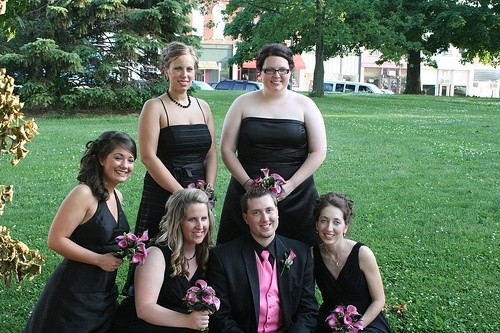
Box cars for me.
[215,80,260,91]
[191,80,214,91]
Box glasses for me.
[261,68,289,75]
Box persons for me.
[310,191,390,333]
[216,42,328,245]
[119,40,216,296]
[23,131,136,333]
[206,185,318,333]
[93,189,213,333]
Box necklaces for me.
[325,239,347,267]
[187,252,196,260]
[167,89,191,108]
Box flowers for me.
[250,168,287,199]
[279,248,297,277]
[187,179,218,209]
[181,279,221,332]
[324,304,364,333]
[115,229,149,266]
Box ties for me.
[261,250,272,277]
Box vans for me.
[322,81,395,95]
